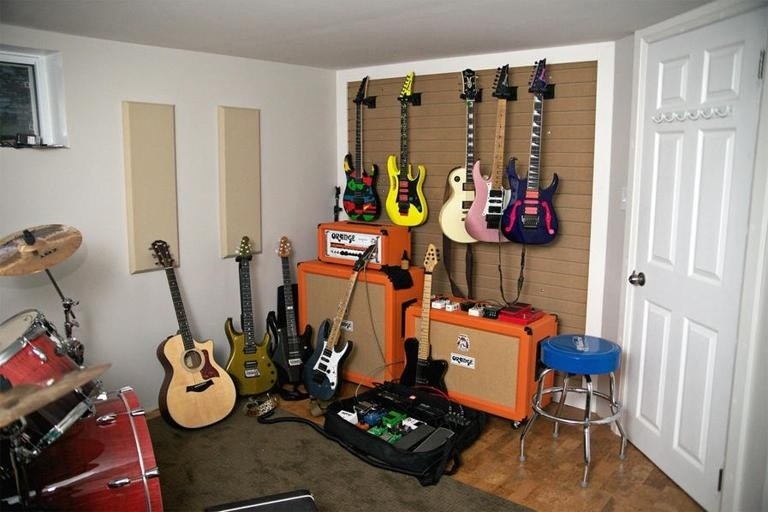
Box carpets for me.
[146,395,529,510]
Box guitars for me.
[343,73,382,222]
[265,235,316,385]
[225,236,279,399]
[149,238,238,430]
[302,246,376,402]
[438,68,489,244]
[384,71,430,229]
[398,243,448,394]
[500,58,559,245]
[464,63,512,244]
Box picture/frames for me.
[0,61,40,139]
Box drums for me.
[0,386,163,511]
[0,308,99,477]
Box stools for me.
[517,333,625,468]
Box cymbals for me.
[0,224,82,276]
[1,363,112,427]
